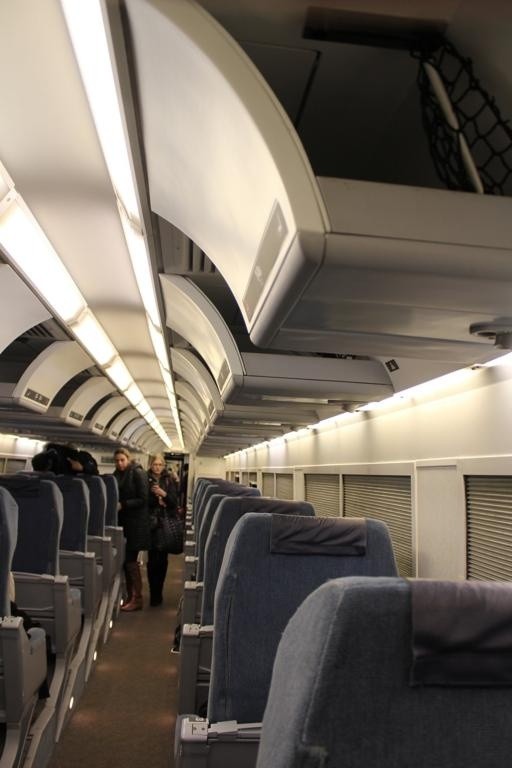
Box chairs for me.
[171,475,510,766]
[0,470,127,768]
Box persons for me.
[167,467,180,495]
[180,462,190,504]
[140,453,184,608]
[45,444,101,475]
[107,448,148,611]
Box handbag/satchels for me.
[160,505,184,555]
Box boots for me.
[121,560,144,611]
